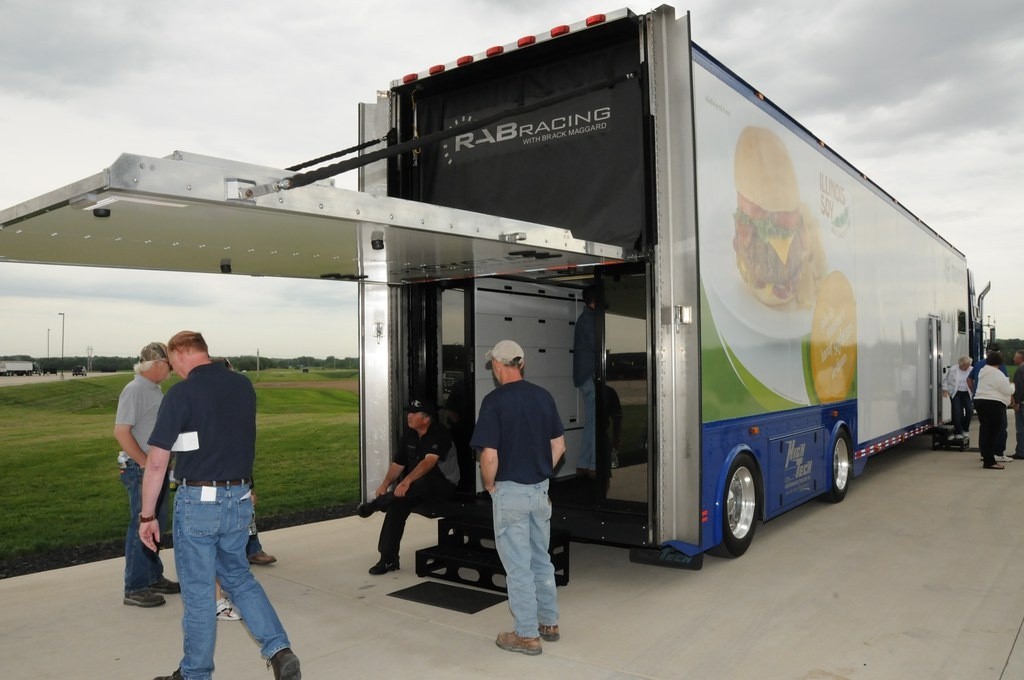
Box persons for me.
[572,287,595,471]
[1006,349,1024,459]
[139,331,302,680]
[115,342,181,607]
[966,343,1013,462]
[357,397,461,576]
[470,340,566,655]
[972,352,1015,470]
[941,356,973,439]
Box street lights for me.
[58,312,65,379]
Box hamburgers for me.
[733,127,803,304]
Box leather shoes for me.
[148,575,181,594]
[124,590,166,608]
[247,550,277,564]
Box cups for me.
[169,468,177,489]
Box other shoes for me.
[1008,454,1024,460]
[984,464,1004,469]
[981,456,983,460]
[995,455,1013,462]
[575,467,596,477]
[956,435,964,439]
[962,431,968,437]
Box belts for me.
[179,477,250,486]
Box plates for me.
[699,192,814,338]
[700,271,809,406]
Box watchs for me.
[139,512,156,523]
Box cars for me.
[72,366,88,378]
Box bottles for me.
[248,507,256,534]
[118,451,128,467]
[611,448,618,468]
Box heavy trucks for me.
[2,3,996,601]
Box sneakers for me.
[368,559,400,574]
[537,624,560,641]
[357,500,379,518]
[496,630,543,654]
[264,647,301,680]
[215,597,242,621]
[153,667,184,680]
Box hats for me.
[485,340,524,364]
[140,342,173,371]
[401,399,434,414]
